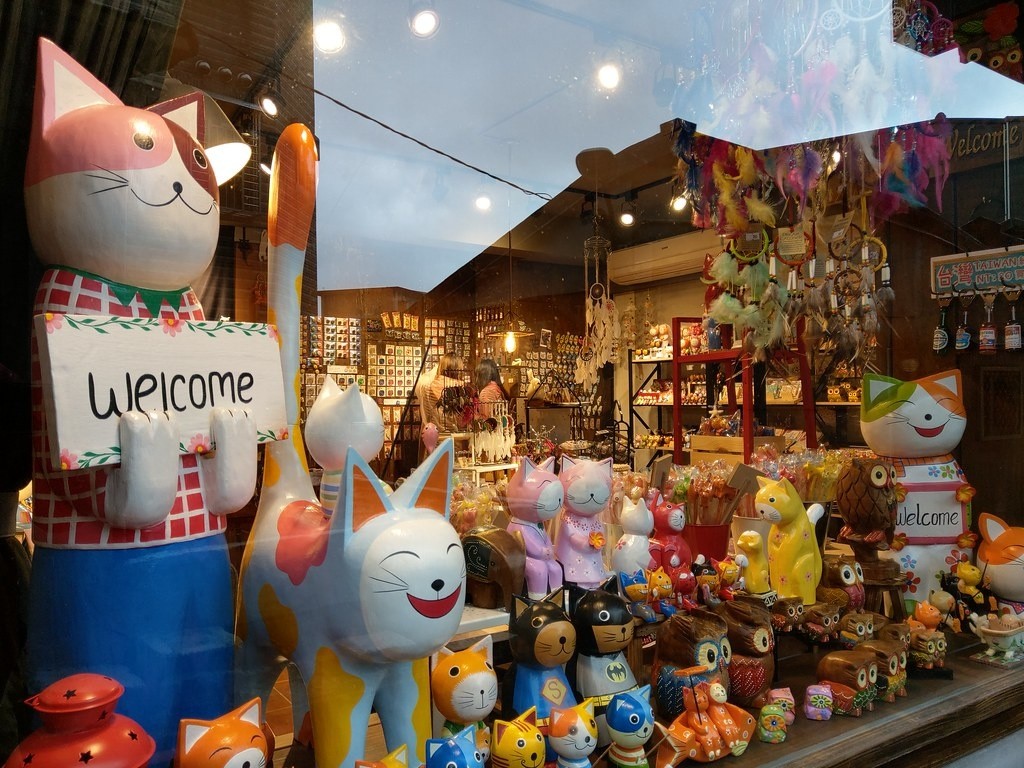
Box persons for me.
[475,357,511,433]
[414,352,466,464]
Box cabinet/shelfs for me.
[628,318,869,472]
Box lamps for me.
[671,179,686,210]
[620,191,638,227]
[407,0,442,40]
[651,54,677,106]
[485,141,536,353]
[580,192,596,224]
[258,49,287,121]
[595,29,625,89]
